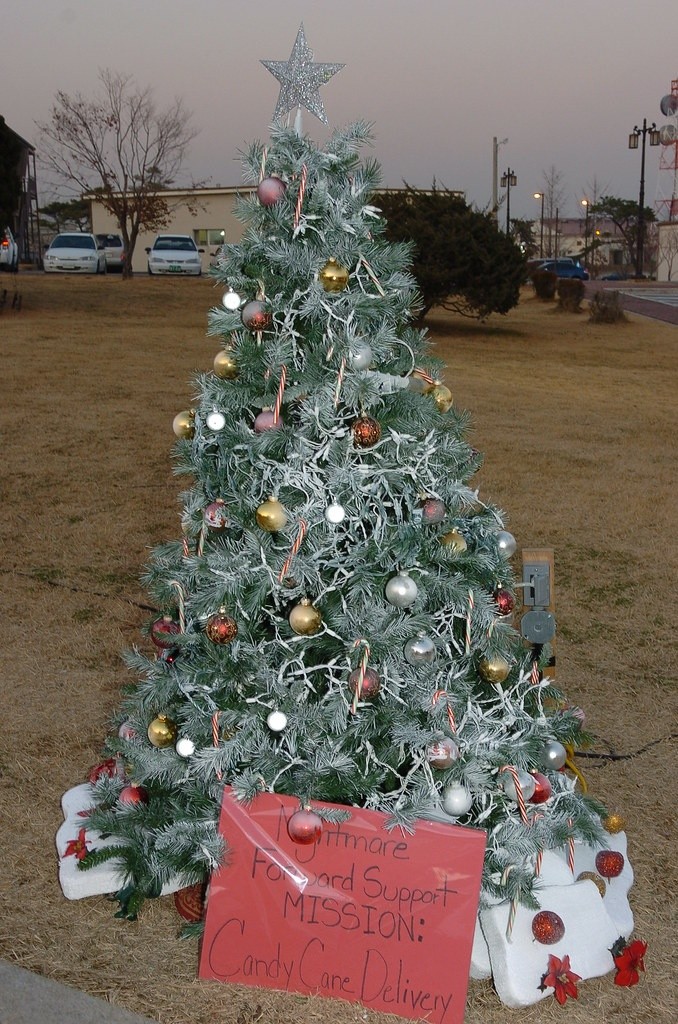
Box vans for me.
[526,258,575,272]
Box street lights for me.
[500,167,517,240]
[533,193,544,259]
[581,200,588,268]
[628,119,661,280]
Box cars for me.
[600,272,628,282]
[209,243,241,267]
[43,232,107,275]
[0,225,19,274]
[91,233,125,272]
[537,262,590,281]
[145,234,205,277]
